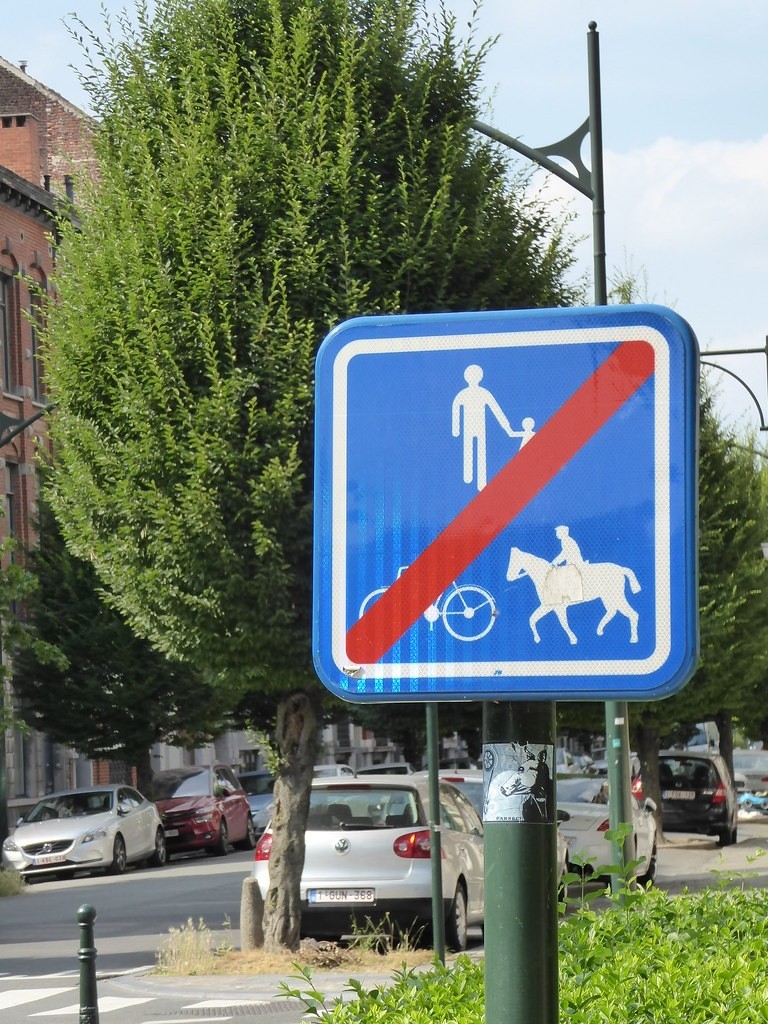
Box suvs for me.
[141,763,256,861]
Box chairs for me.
[322,804,353,830]
[658,764,673,786]
[692,766,711,788]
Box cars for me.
[2,784,166,883]
[556,747,594,774]
[686,724,715,752]
[355,762,414,776]
[632,751,745,845]
[252,774,483,952]
[629,751,641,777]
[733,750,768,814]
[421,757,475,770]
[238,770,275,839]
[313,764,354,777]
[556,773,658,889]
[381,769,570,903]
[589,759,608,773]
